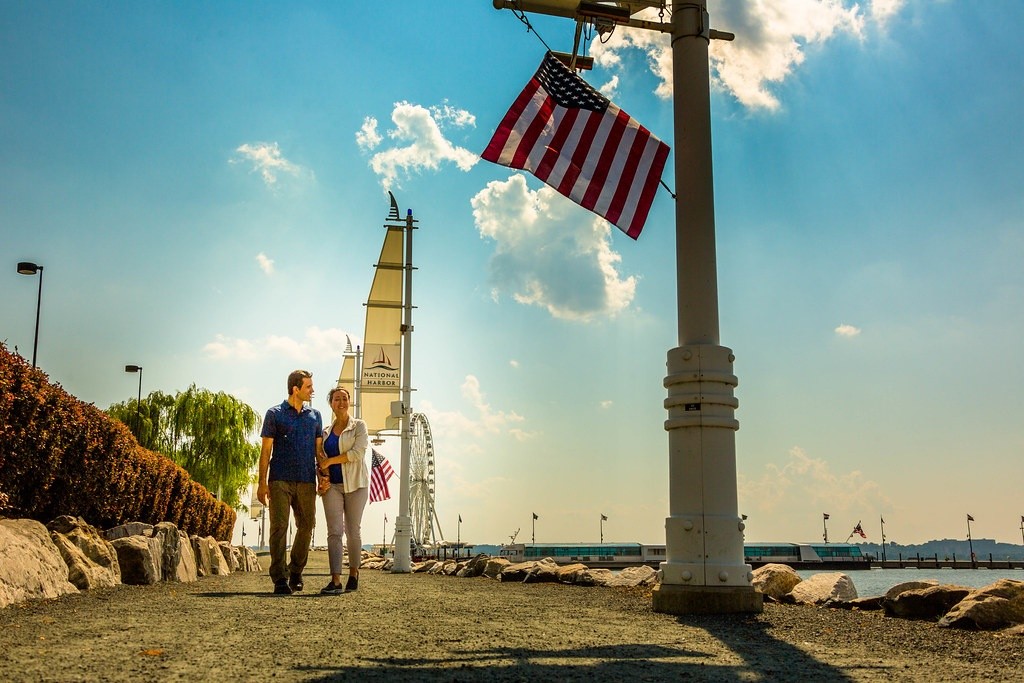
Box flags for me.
[881,518,884,523]
[855,525,866,538]
[459,516,462,522]
[601,515,607,521]
[385,517,388,522]
[534,514,538,520]
[370,450,394,504]
[742,515,747,520]
[824,514,829,519]
[481,51,670,240]
[968,515,973,521]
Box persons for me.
[257,371,329,594]
[316,387,369,594]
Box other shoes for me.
[289,569,304,591]
[345,569,359,592]
[274,582,293,594]
[321,581,342,594]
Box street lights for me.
[17,260,43,366]
[124,365,142,435]
